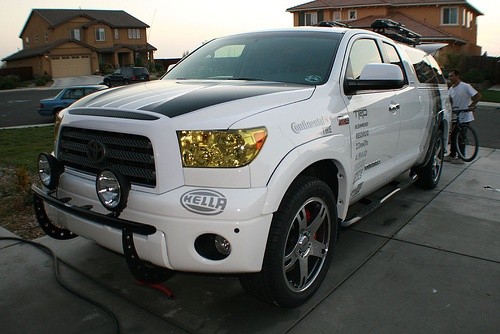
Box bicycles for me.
[451,107,479,163]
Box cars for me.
[37,84,109,122]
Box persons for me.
[442,69,481,164]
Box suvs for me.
[102,66,150,89]
[28,18,453,310]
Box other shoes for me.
[457,158,463,164]
[443,155,452,162]
